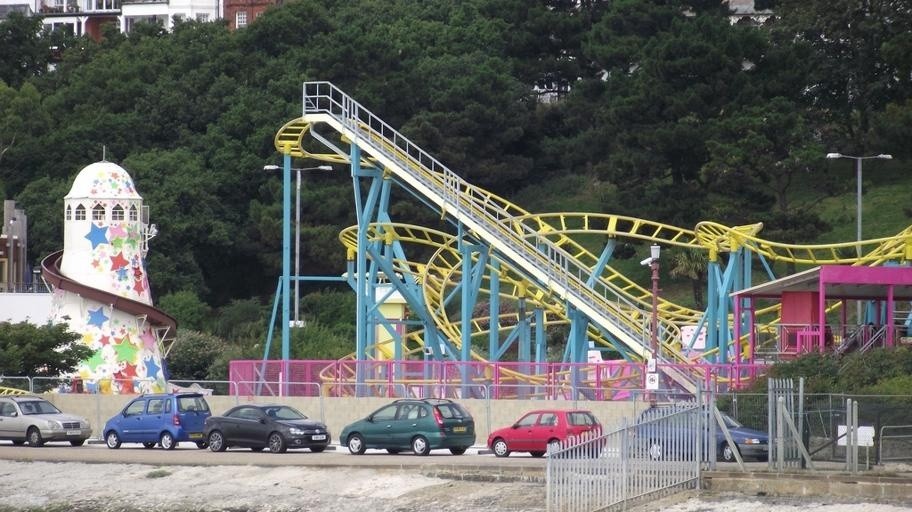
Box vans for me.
[101,390,219,449]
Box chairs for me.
[240,409,279,420]
[546,416,574,425]
[397,406,426,418]
[152,401,195,411]
[3,404,42,414]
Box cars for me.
[0,393,95,447]
[201,401,334,456]
[485,407,607,461]
[630,401,777,465]
[337,394,480,456]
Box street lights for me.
[259,162,335,329]
[825,151,896,326]
[647,241,665,408]
